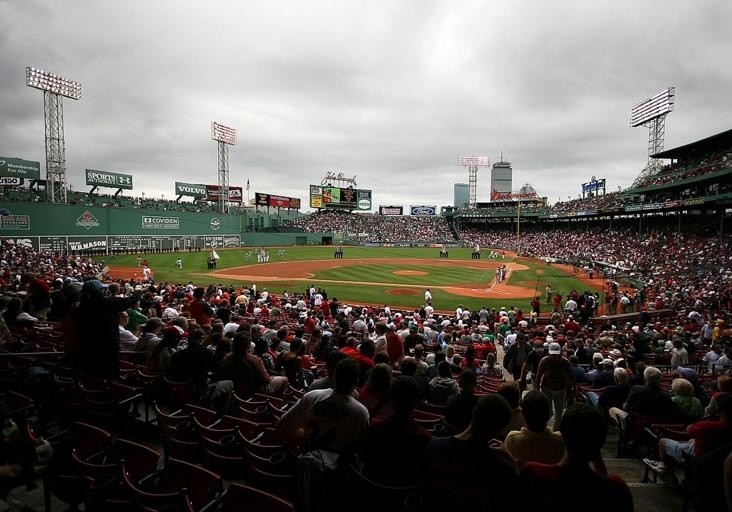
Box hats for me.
[548,342,561,355]
[610,349,621,356]
[599,358,614,366]
[83,279,110,292]
[677,365,696,380]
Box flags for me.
[246,180,250,189]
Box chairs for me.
[0,316,731,511]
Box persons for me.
[459,148,732,239]
[1,240,732,512]
[283,209,457,245]
